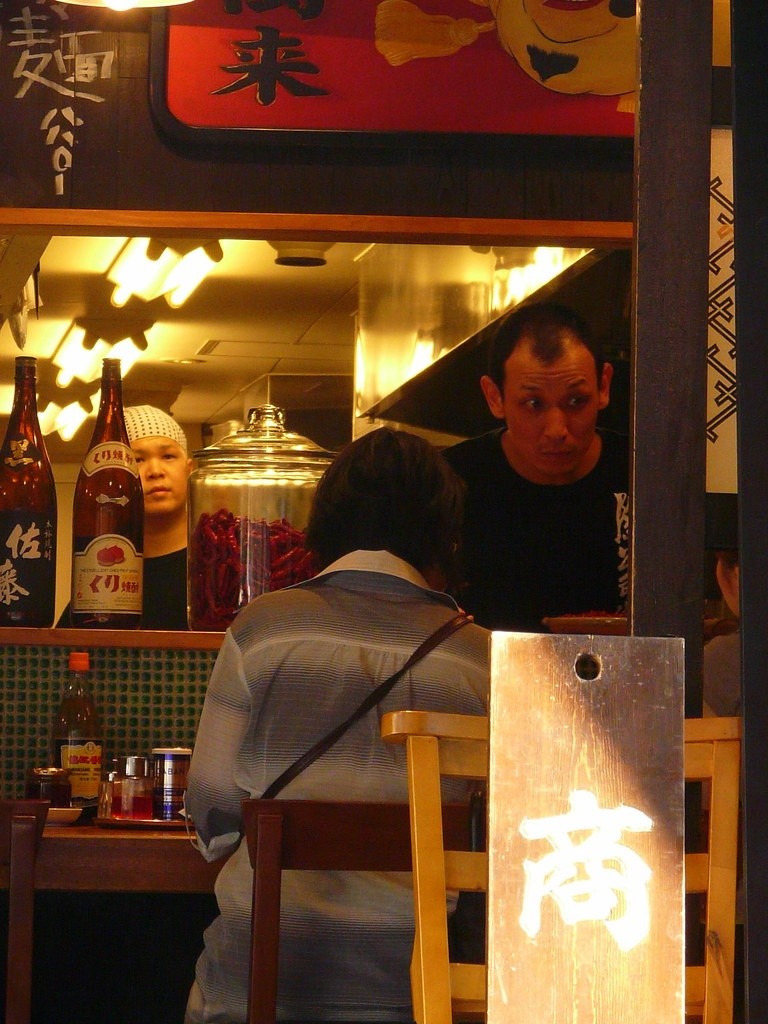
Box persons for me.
[431,298,636,643]
[114,400,201,626]
[698,527,746,730]
[185,422,505,1024]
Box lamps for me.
[29,236,225,441]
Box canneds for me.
[150,748,193,819]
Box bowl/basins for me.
[44,808,83,827]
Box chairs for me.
[239,793,472,1024]
[379,711,750,1024]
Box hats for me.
[124,403,188,452]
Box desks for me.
[31,825,245,895]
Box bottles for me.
[51,650,102,826]
[186,402,340,633]
[152,747,191,820]
[72,358,144,631]
[111,756,156,822]
[25,766,72,809]
[97,772,118,818]
[2,355,58,627]
